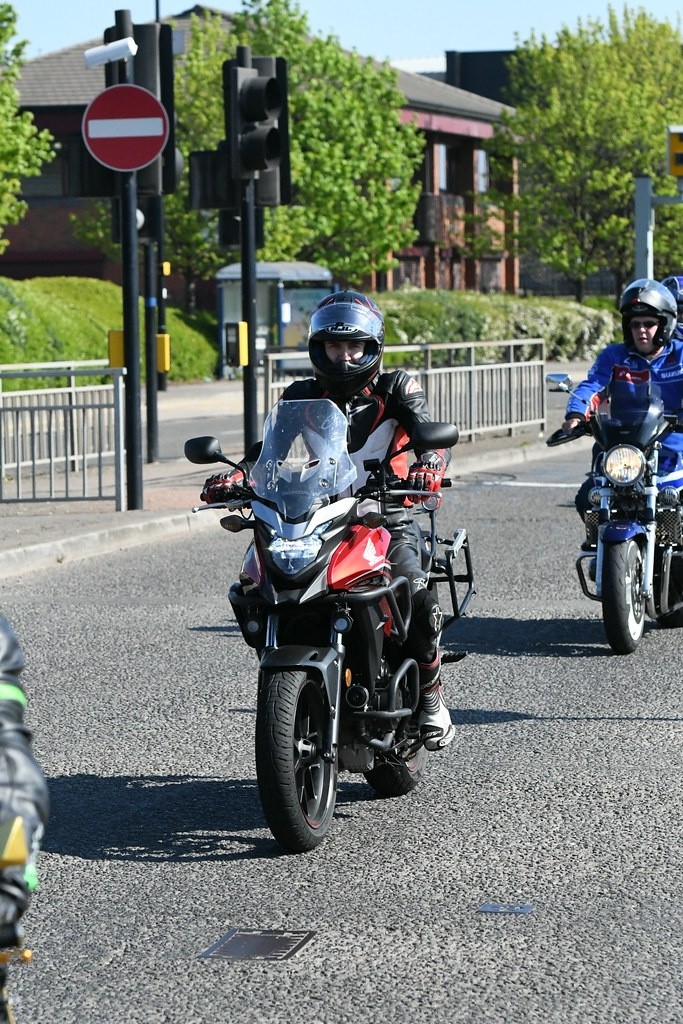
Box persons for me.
[563,274,683,555]
[202,289,458,752]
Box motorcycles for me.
[183,394,477,853]
[544,373,683,654]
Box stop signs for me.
[82,83,170,172]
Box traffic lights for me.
[230,66,285,181]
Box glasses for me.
[627,320,660,329]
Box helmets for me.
[621,279,677,350]
[307,290,384,398]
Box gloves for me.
[407,447,451,503]
[201,463,253,509]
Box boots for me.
[417,648,454,750]
